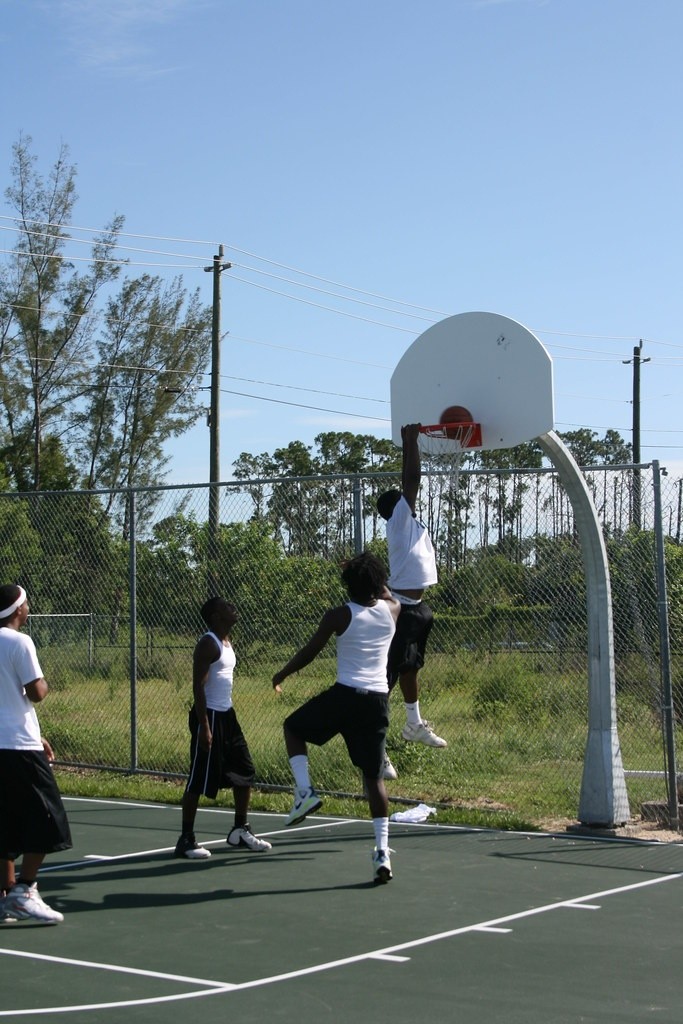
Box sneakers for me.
[227,823,272,851]
[402,719,446,748]
[175,832,211,858]
[284,786,322,825]
[371,845,396,882]
[2,882,64,922]
[383,752,398,781]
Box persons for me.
[174,597,272,858]
[377,423,448,779]
[0,583,72,923]
[272,551,401,883]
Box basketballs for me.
[439,406,474,440]
